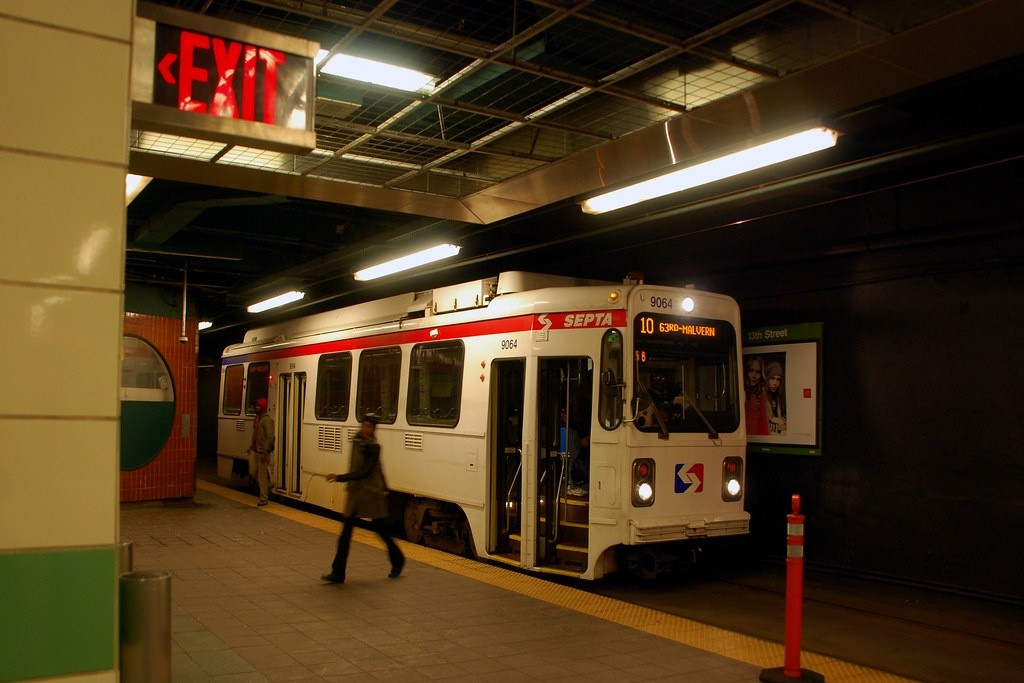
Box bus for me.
[217,271,754,581]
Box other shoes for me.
[257,500,267,506]
[388,560,406,578]
[320,573,345,584]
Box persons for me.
[648,368,681,413]
[568,368,593,496]
[744,356,787,435]
[320,417,405,584]
[505,364,561,444]
[246,398,275,505]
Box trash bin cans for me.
[119,538,135,575]
[119,569,173,683]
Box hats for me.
[763,361,782,380]
[256,398,268,414]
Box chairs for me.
[333,395,349,408]
